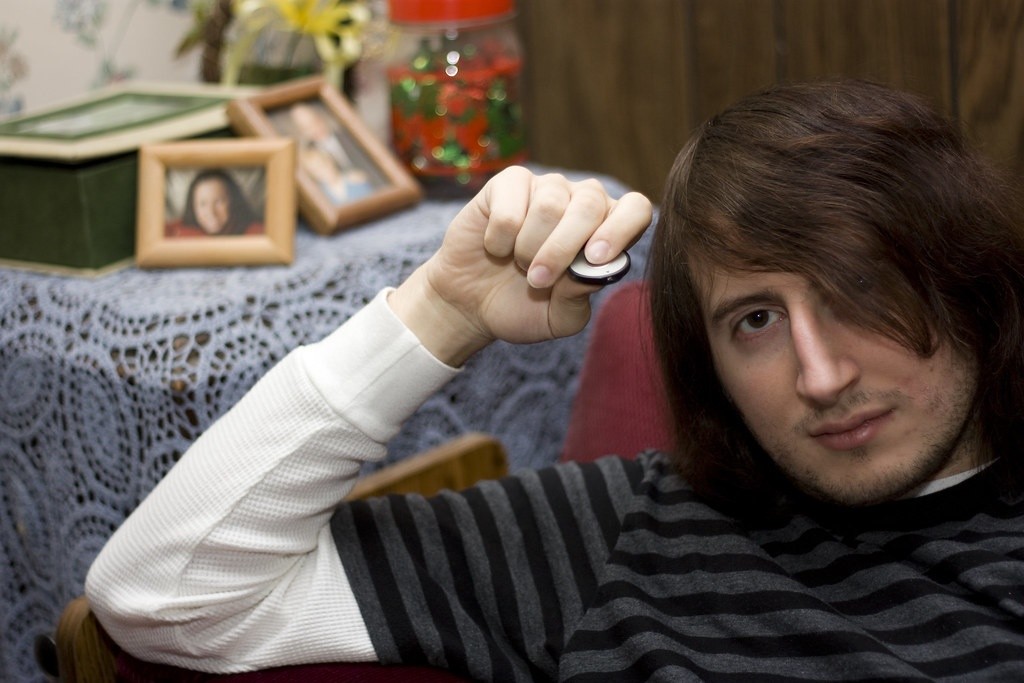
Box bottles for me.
[386,0,526,195]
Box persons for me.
[294,103,380,205]
[82,76,1024,683]
[167,171,263,237]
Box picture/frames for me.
[229,75,421,235]
[137,137,298,268]
[0,78,258,159]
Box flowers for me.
[177,1,370,68]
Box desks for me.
[1,156,659,683]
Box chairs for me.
[36,431,512,683]
